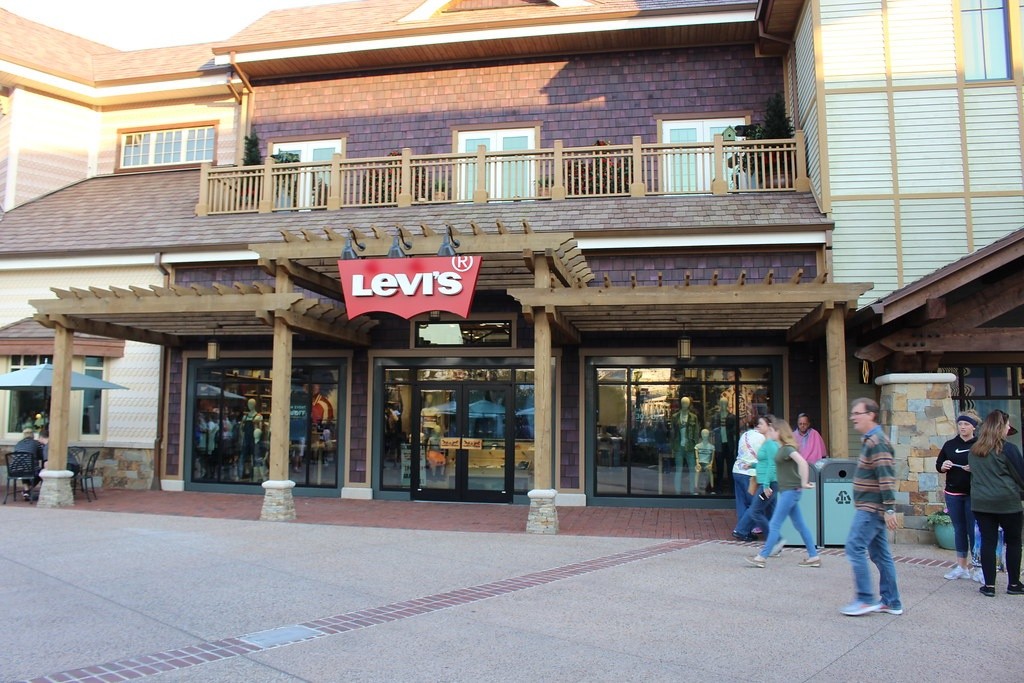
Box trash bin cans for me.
[777,461,820,548]
[815,457,858,548]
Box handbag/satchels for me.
[747,477,759,495]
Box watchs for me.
[886,509,894,514]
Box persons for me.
[239,398,263,477]
[38,430,80,478]
[968,410,1024,597]
[840,398,903,615]
[732,414,786,556]
[383,403,403,468]
[743,419,821,567]
[12,428,43,500]
[670,397,700,495]
[790,413,827,465]
[710,397,738,494]
[427,424,446,481]
[693,429,716,495]
[196,411,241,480]
[936,409,987,584]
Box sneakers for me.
[973,568,985,585]
[875,603,903,614]
[840,600,880,615]
[945,565,970,580]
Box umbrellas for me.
[196,383,246,399]
[0,357,130,431]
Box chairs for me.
[3,446,100,504]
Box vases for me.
[935,523,956,550]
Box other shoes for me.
[732,530,758,542]
[773,538,785,556]
[752,528,762,533]
[979,586,995,596]
[22,491,30,500]
[1007,581,1024,594]
[745,557,764,568]
[799,558,820,566]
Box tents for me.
[423,399,535,439]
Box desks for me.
[659,450,676,495]
[288,443,336,485]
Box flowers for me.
[925,507,953,527]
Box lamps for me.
[428,309,440,321]
[438,225,461,256]
[677,322,690,361]
[341,229,366,260]
[206,329,219,362]
[388,227,413,257]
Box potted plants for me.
[738,93,796,187]
[240,123,262,211]
[271,148,299,211]
[429,180,448,201]
[532,177,554,198]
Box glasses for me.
[849,411,868,416]
